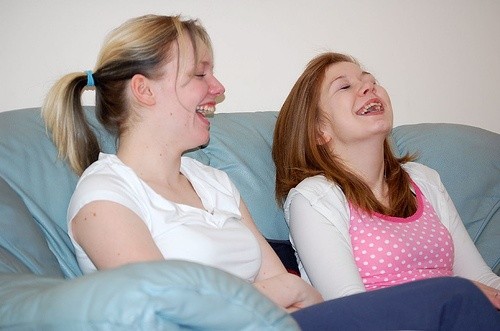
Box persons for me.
[41,14,500,331]
[271,51,500,315]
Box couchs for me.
[0,106,500,331]
